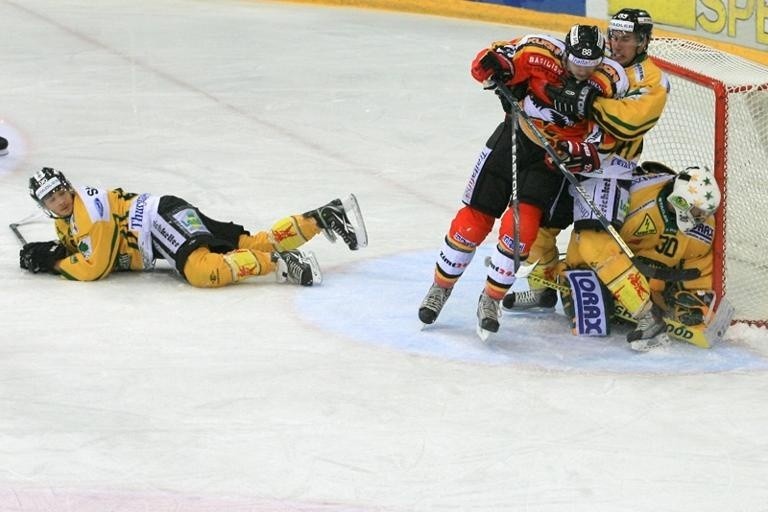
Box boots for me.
[271,249,312,286]
[303,199,358,250]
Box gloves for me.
[471,48,515,89]
[545,74,602,120]
[544,140,600,172]
[20,241,65,273]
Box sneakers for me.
[627,310,665,342]
[419,282,453,324]
[477,289,502,332]
[503,288,557,309]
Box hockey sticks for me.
[497,76,700,281]
[484,257,734,347]
[10,220,37,248]
[511,95,540,279]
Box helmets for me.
[607,8,653,47]
[565,24,605,72]
[29,167,75,219]
[666,165,721,233]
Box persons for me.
[503,7,670,312]
[553,162,723,352]
[419,25,629,340]
[0,137,9,156]
[18,166,368,290]
